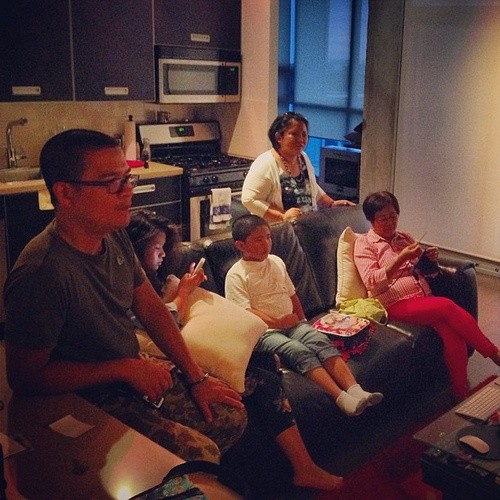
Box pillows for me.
[335,226,367,306]
[133,274,272,392]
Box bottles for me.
[125,115,136,160]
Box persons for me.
[354,191,500,396]
[225,214,383,416]
[2,128,247,464]
[242,111,358,220]
[125,208,344,490]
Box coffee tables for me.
[411,376,500,500]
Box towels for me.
[208,187,232,230]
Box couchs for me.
[164,203,480,490]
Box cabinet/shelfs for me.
[0,0,242,101]
[1,192,56,276]
[132,175,182,238]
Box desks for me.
[0,343,247,500]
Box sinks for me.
[0,168,43,183]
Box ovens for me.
[179,190,251,248]
[156,57,242,104]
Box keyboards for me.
[453,386,500,424]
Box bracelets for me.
[329,201,333,208]
[192,370,208,385]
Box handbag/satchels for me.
[338,298,389,326]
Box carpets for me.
[310,374,498,500]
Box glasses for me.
[146,366,184,408]
[64,174,139,193]
[275,112,303,132]
[373,214,399,226]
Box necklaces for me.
[281,156,303,182]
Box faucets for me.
[5,116,29,169]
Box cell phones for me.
[192,258,205,275]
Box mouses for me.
[458,435,489,454]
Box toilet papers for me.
[122,120,136,160]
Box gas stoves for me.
[136,121,255,194]
